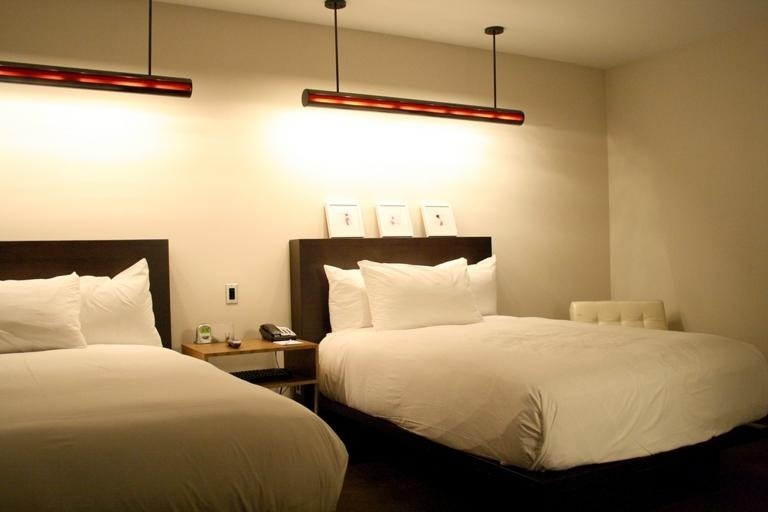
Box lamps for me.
[301,0,525,127]
[0,0,194,99]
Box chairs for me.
[568,300,668,331]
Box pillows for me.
[322,263,373,335]
[0,270,87,354]
[78,257,163,347]
[467,254,498,318]
[356,257,482,336]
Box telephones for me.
[259,323,297,343]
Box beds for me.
[0,238,351,512]
[289,235,768,512]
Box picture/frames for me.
[375,204,413,238]
[324,203,365,237]
[420,205,458,236]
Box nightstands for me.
[181,338,322,416]
